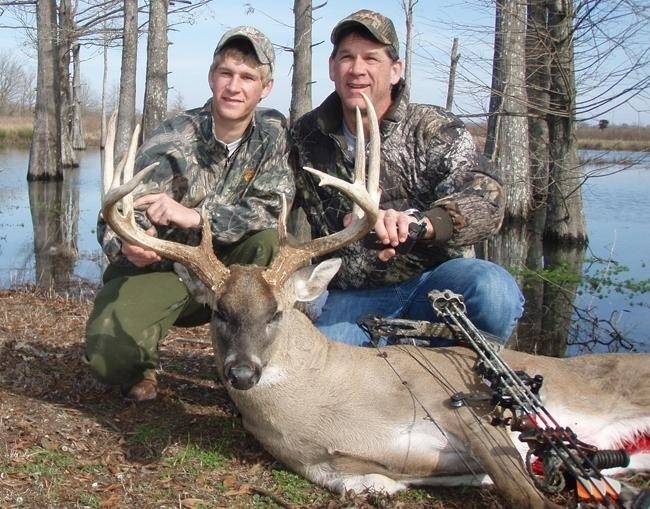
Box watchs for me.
[404,208,427,238]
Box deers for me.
[100,90,650,502]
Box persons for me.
[289,9,525,349]
[81,26,297,402]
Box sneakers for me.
[120,369,159,401]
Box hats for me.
[331,9,399,53]
[214,26,275,65]
